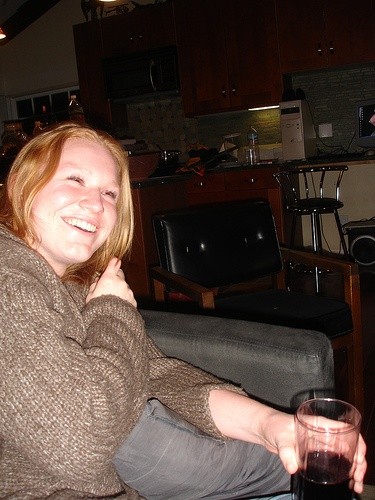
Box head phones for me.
[281,88,305,102]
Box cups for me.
[290,398,362,500]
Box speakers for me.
[346,228,375,292]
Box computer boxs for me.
[279,100,312,162]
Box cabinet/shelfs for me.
[72,0,375,309]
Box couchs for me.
[139,310,335,420]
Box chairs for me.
[150,197,365,420]
[274,166,350,261]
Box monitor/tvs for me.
[355,98,375,156]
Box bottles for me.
[246,125,260,166]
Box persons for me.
[0,122,367,500]
